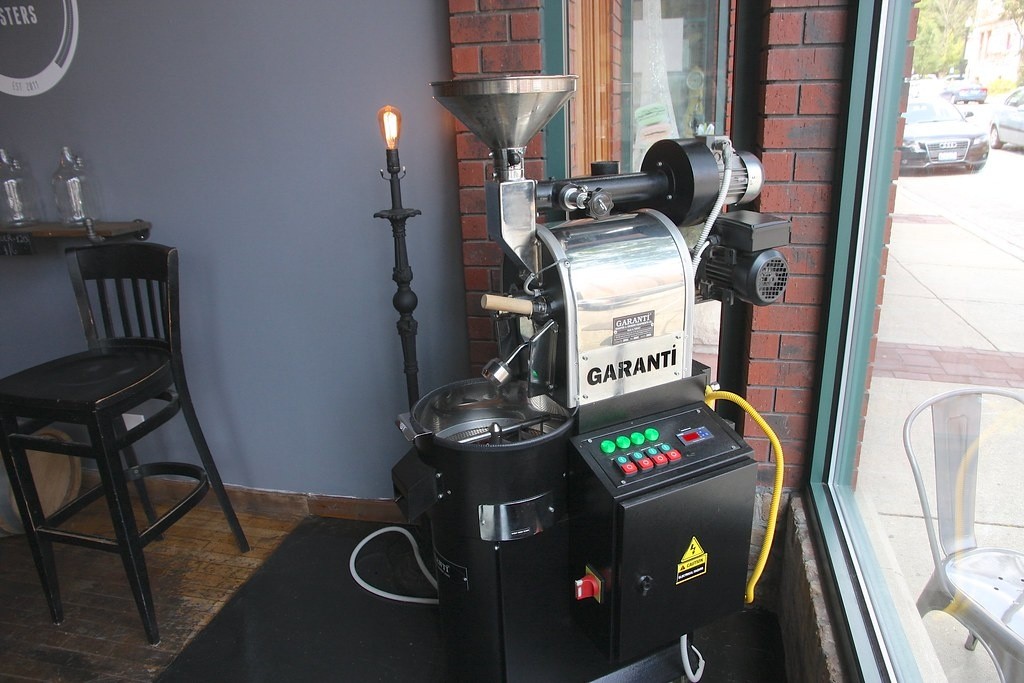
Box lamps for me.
[374,107,437,596]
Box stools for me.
[0,240,250,647]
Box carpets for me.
[156,514,788,683]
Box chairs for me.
[902,385,1024,683]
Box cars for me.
[900,75,989,171]
[989,85,1024,149]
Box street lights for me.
[959,16,973,80]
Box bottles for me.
[0,148,42,229]
[49,145,99,228]
